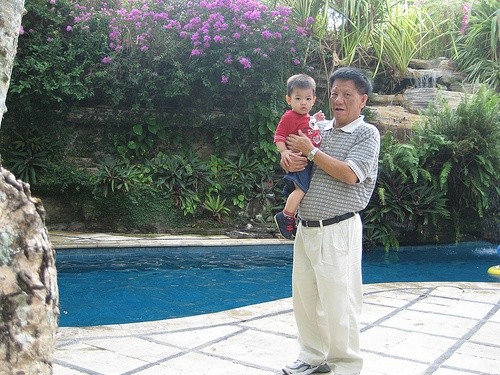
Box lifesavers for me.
[487,264,500,275]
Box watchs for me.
[306,147,321,162]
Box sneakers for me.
[282,359,332,375]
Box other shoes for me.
[292,216,298,238]
[274,212,294,240]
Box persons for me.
[273,73,328,242]
[282,67,381,374]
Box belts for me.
[295,212,358,227]
